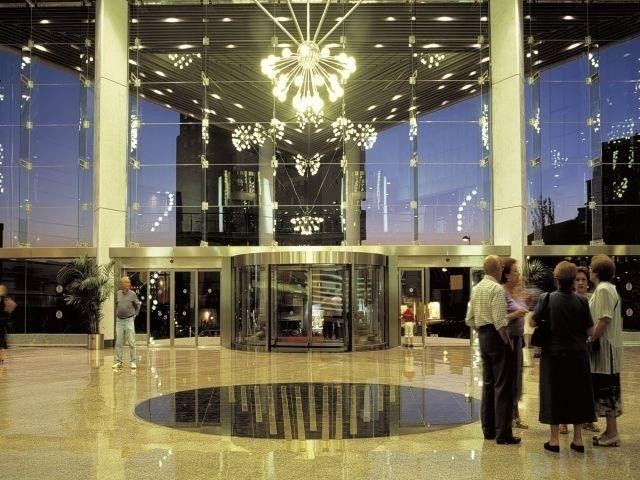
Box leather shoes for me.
[592,433,603,440]
[592,435,621,447]
[570,441,585,452]
[496,435,521,444]
[484,434,496,440]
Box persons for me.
[529,259,595,453]
[558,266,599,433]
[586,253,622,447]
[465,254,520,443]
[400,303,416,348]
[500,258,539,428]
[320,281,353,340]
[0,283,20,364]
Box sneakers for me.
[112,361,123,368]
[514,420,528,429]
[404,344,414,348]
[129,360,137,370]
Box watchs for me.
[111,277,142,369]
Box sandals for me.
[559,424,568,434]
[582,423,600,432]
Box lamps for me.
[209,309,214,321]
[249,0,364,114]
[167,51,445,236]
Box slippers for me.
[543,442,560,453]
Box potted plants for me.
[56,252,121,349]
[522,260,554,311]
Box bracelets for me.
[587,336,595,343]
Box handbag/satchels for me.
[529,292,551,348]
